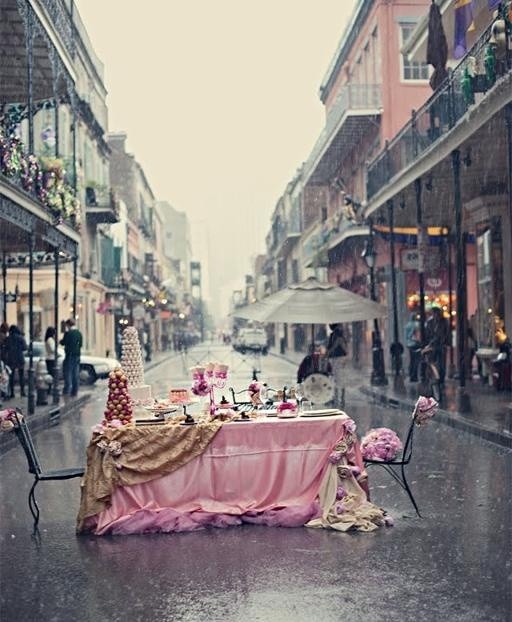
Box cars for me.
[233,328,269,354]
[13,341,121,384]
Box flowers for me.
[361,427,401,462]
[3,136,80,226]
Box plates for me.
[301,409,339,417]
[304,373,334,405]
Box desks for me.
[77,403,372,533]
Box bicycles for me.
[415,348,441,402]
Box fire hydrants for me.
[35,360,53,405]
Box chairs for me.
[9,407,86,529]
[358,394,424,515]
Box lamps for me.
[374,175,434,223]
[461,151,473,168]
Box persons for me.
[3,325,30,398]
[116,326,123,359]
[141,328,150,360]
[401,303,481,382]
[44,327,59,395]
[160,332,204,351]
[59,318,83,395]
[326,323,347,406]
[297,344,334,383]
[0,323,10,363]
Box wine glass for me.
[146,404,178,419]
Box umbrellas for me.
[426,0,450,94]
[226,276,391,371]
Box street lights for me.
[360,240,388,385]
